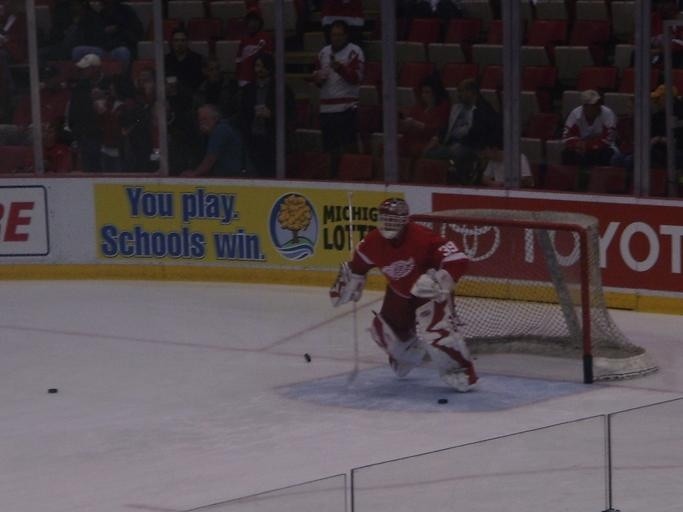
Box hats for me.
[75,54,100,70]
[580,90,602,105]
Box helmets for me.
[378,199,408,240]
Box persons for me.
[329,197,479,392]
[1,2,682,193]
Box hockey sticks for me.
[343,194,360,387]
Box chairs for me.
[0,1,682,200]
